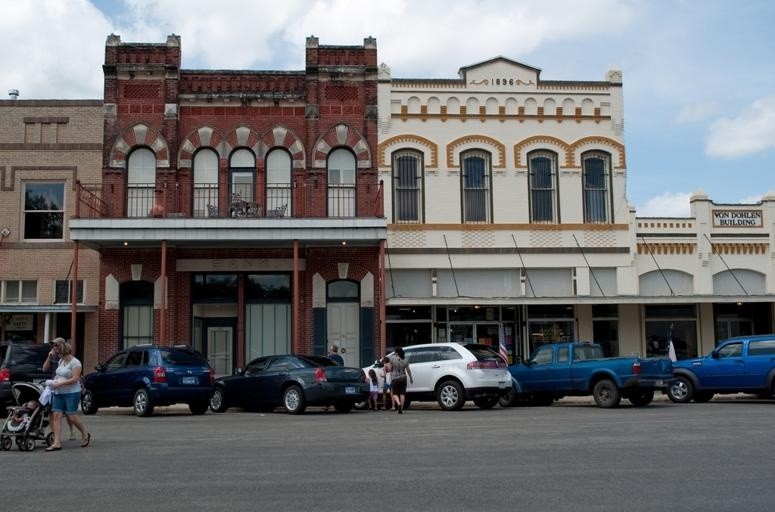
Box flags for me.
[499,341,509,366]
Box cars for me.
[210,354,371,413]
[668,334,775,404]
[80,343,215,415]
[356,340,512,411]
[1,340,60,417]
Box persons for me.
[366,369,378,410]
[42,336,77,441]
[46,342,90,452]
[323,344,344,409]
[380,356,397,411]
[390,346,413,414]
[11,400,37,422]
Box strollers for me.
[0,380,57,451]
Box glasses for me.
[52,344,59,347]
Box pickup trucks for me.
[500,340,677,408]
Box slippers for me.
[45,446,62,451]
[81,433,90,447]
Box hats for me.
[51,338,65,343]
